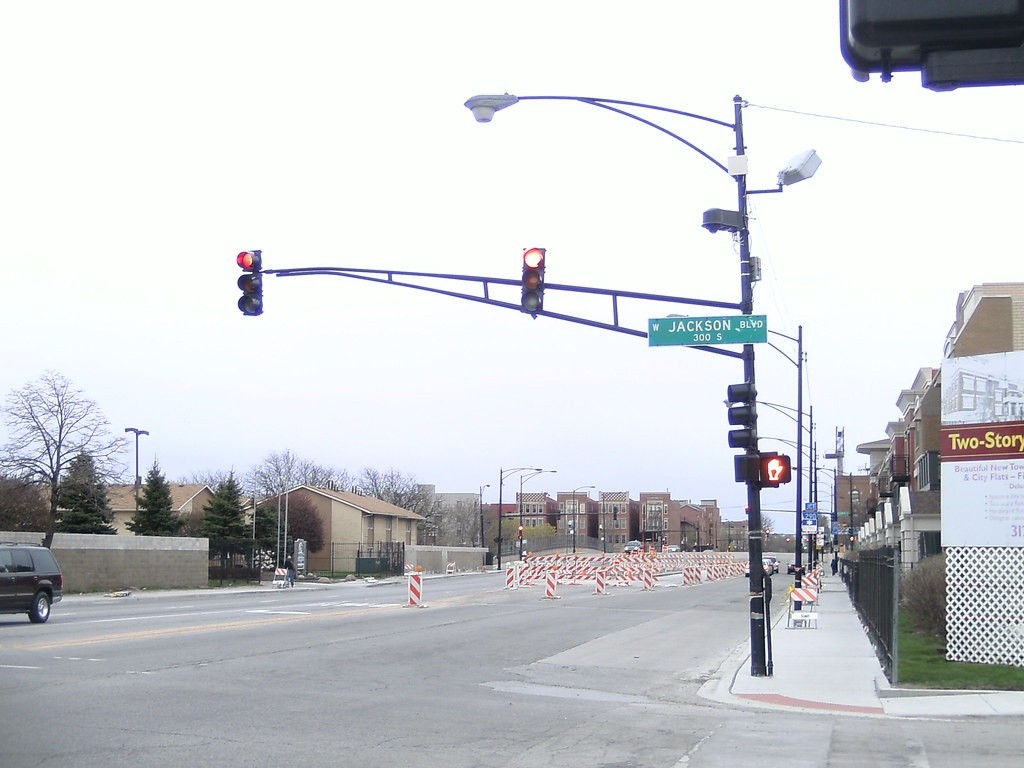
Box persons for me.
[831,559,837,575]
[284,555,296,588]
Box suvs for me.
[0,542,65,623]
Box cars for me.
[762,556,780,572]
[667,545,681,552]
[624,539,642,554]
[744,559,773,577]
[786,561,807,575]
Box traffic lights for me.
[726,380,758,451]
[522,247,545,315]
[648,315,768,346]
[237,250,264,317]
[761,455,791,484]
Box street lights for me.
[517,469,557,561]
[124,426,149,513]
[479,483,490,546]
[572,484,596,555]
[497,464,543,570]
[461,78,822,570]
